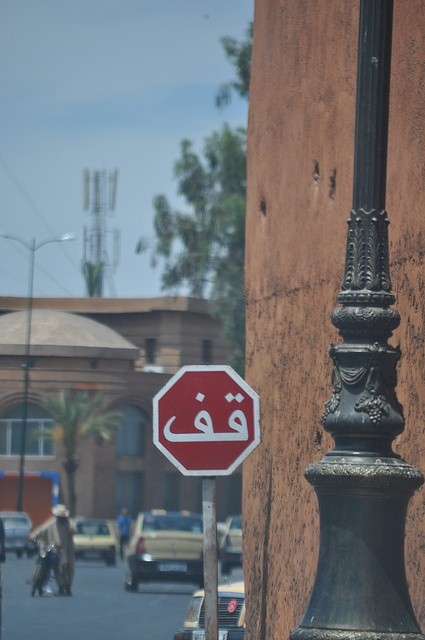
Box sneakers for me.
[56,589,65,596]
[66,589,71,596]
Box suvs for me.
[0,513,32,558]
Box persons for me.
[32,504,79,596]
[116,508,130,556]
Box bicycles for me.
[26,538,64,596]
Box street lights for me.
[0,232,77,558]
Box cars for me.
[220,515,243,573]
[124,509,203,592]
[175,580,245,640]
[72,520,115,565]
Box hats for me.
[51,505,70,518]
